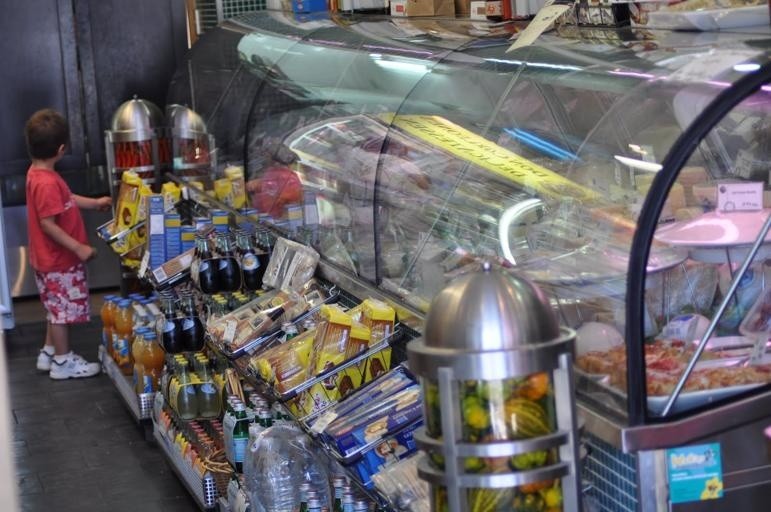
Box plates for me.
[574,190,771,416]
[647,6,770,30]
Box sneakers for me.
[37,348,53,371]
[50,354,100,379]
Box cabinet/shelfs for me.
[168,14,771,511]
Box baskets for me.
[207,281,430,512]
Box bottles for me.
[101,224,387,512]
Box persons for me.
[24,108,112,382]
